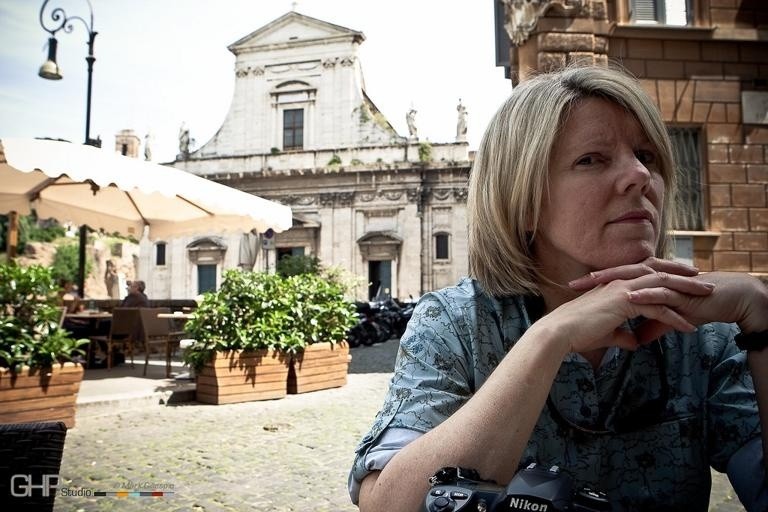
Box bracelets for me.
[732,329,768,354]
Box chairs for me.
[7,301,191,379]
[0,419,67,511]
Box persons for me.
[98,280,150,367]
[455,104,469,136]
[54,278,83,312]
[344,62,768,512]
[178,129,192,153]
[143,134,152,159]
[404,110,418,136]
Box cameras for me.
[414,457,615,512]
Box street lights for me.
[37,0,100,300]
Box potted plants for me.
[0,258,92,428]
[177,249,371,408]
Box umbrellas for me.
[0,138,295,246]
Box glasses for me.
[546,337,697,435]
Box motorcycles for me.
[347,293,415,348]
